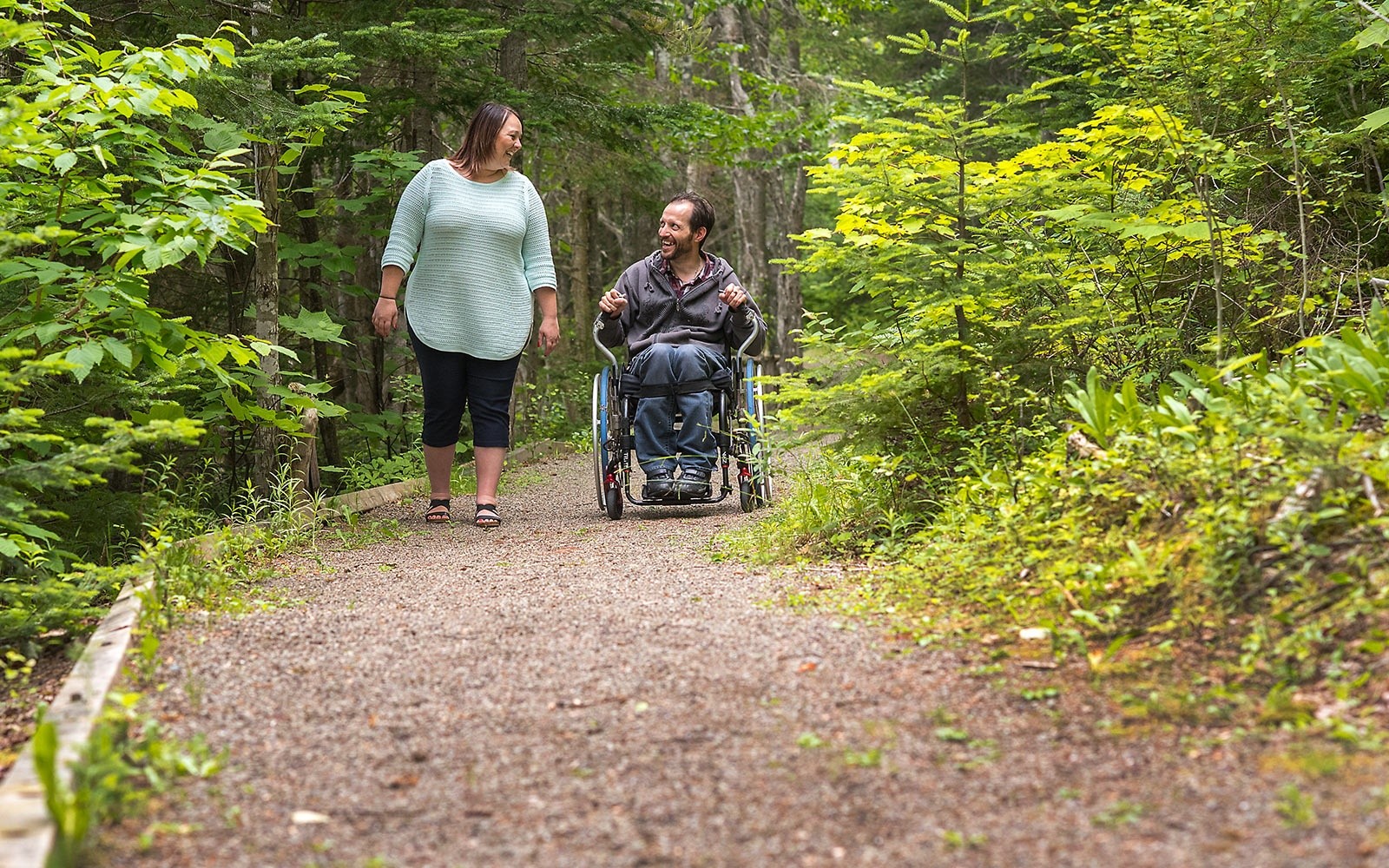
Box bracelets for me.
[379,296,394,301]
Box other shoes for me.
[646,468,675,496]
[674,467,712,497]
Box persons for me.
[372,101,561,527]
[599,191,769,502]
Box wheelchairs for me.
[591,289,774,521]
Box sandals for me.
[474,504,501,527]
[425,499,452,523]
